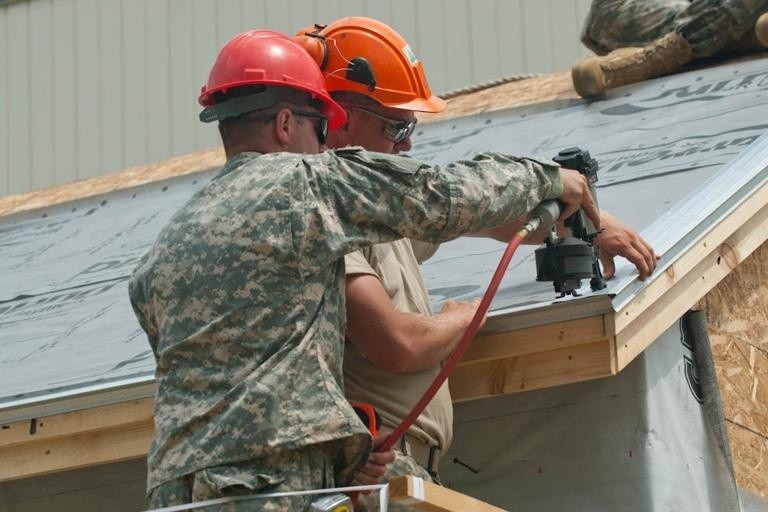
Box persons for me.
[296,15,664,512]
[126,28,603,512]
[570,1,767,98]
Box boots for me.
[572,29,691,97]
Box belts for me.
[382,425,442,475]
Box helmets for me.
[198,16,448,133]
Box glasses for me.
[348,106,417,144]
[293,110,329,144]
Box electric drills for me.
[527,147,606,299]
